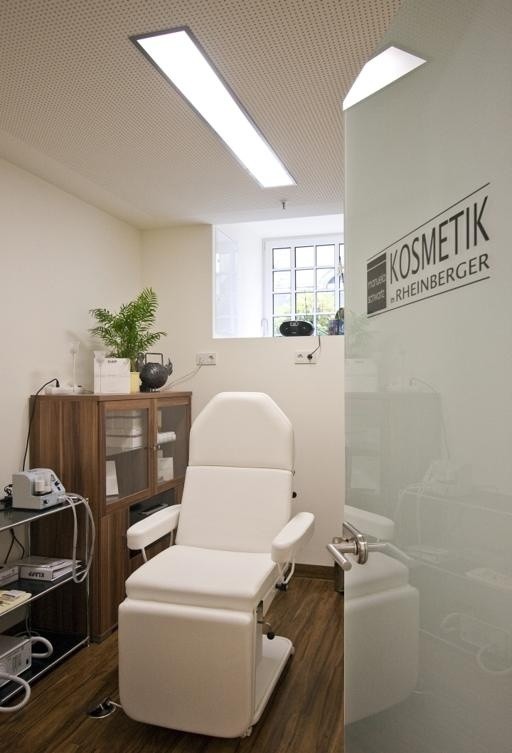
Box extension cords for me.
[44,384,85,395]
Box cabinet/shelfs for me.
[0,494,91,711]
[28,389,194,645]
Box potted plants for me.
[86,285,167,392]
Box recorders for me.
[279,319,315,336]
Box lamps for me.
[128,23,300,192]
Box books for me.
[0,555,82,588]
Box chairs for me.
[116,390,316,739]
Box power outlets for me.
[193,350,222,369]
[293,349,321,366]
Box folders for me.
[4,555,83,582]
